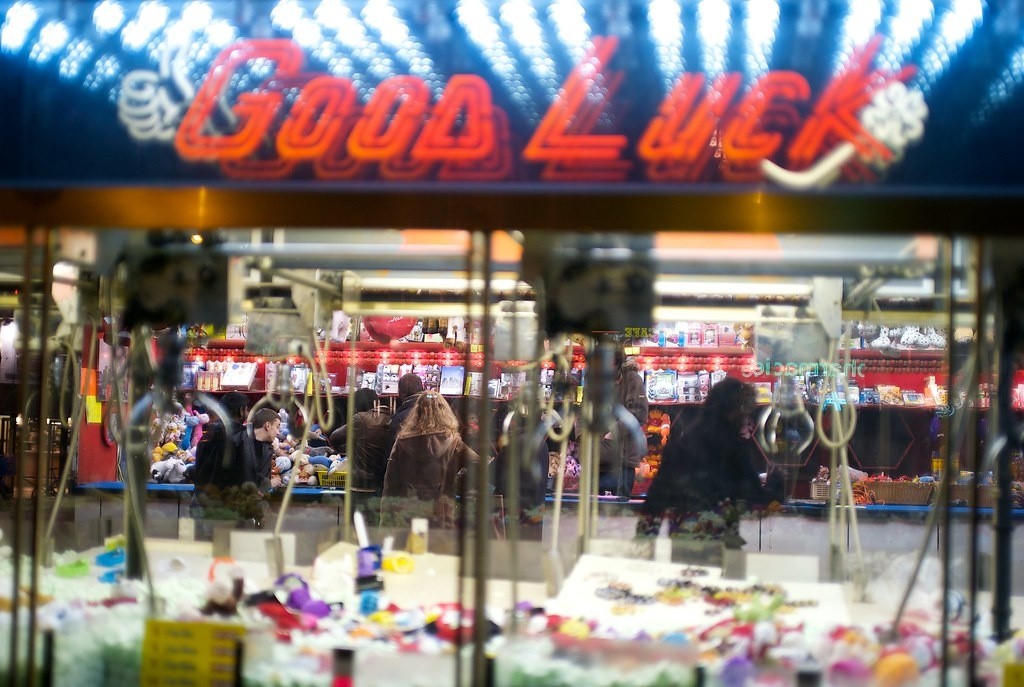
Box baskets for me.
[865,480,933,504]
[940,484,995,508]
[316,470,347,487]
[809,481,829,499]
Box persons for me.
[635,377,790,581]
[387,373,426,461]
[330,387,393,527]
[487,401,549,543]
[238,408,283,502]
[190,391,249,542]
[379,390,480,557]
[570,340,649,516]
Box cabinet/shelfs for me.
[13,423,62,453]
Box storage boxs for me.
[645,370,726,403]
[195,372,220,391]
[754,382,771,403]
[631,322,737,348]
[221,363,258,390]
[902,392,926,406]
[182,365,198,388]
[978,384,1024,408]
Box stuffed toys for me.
[546,408,672,496]
[466,412,479,438]
[144,389,350,483]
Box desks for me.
[47,546,547,622]
[175,390,1024,497]
[551,554,1023,638]
[74,481,1024,560]
